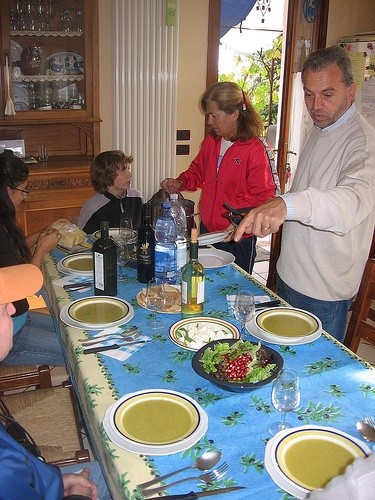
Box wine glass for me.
[268,369,301,436]
[145,279,166,330]
[120,218,134,258]
[114,240,129,281]
[233,288,255,340]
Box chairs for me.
[343,258,375,354]
[0,363,91,469]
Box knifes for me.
[83,341,151,355]
[144,482,247,500]
[224,201,244,219]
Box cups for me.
[38,145,48,161]
[8,0,83,33]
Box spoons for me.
[78,327,139,342]
[356,420,375,442]
[137,451,222,490]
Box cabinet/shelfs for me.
[0,0,100,239]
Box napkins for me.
[81,327,153,362]
[52,275,94,293]
[225,294,271,315]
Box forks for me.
[365,416,375,430]
[142,461,231,497]
[81,329,143,347]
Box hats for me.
[0,264,43,304]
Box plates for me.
[10,40,23,62]
[136,285,181,315]
[167,306,373,500]
[55,240,135,330]
[187,229,235,269]
[103,388,209,456]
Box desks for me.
[31,234,375,500]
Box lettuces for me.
[198,339,276,384]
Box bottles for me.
[94,194,205,315]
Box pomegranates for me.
[222,352,251,381]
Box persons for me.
[233,45,375,343]
[72,150,143,235]
[0,148,66,367]
[0,264,114,500]
[160,81,277,275]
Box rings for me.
[263,227,269,231]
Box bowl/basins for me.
[191,338,284,394]
[222,207,258,226]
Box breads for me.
[52,222,86,248]
[138,283,182,312]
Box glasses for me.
[9,185,29,199]
